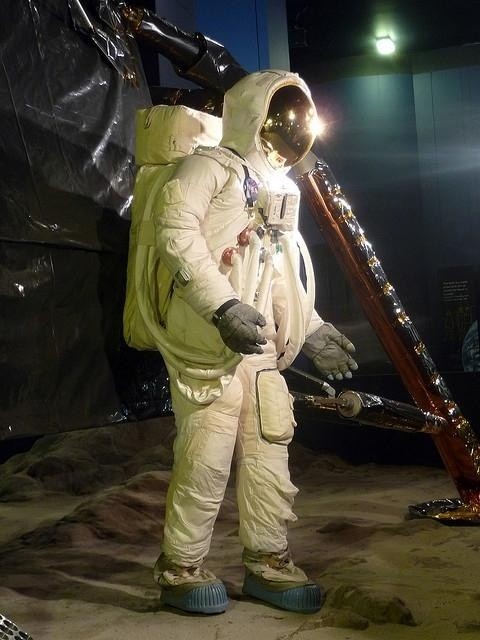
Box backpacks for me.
[123,105,248,351]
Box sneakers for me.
[162,579,229,613]
[244,572,321,613]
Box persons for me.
[152,67,362,615]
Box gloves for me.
[212,299,267,354]
[302,322,358,380]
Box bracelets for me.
[211,299,239,327]
